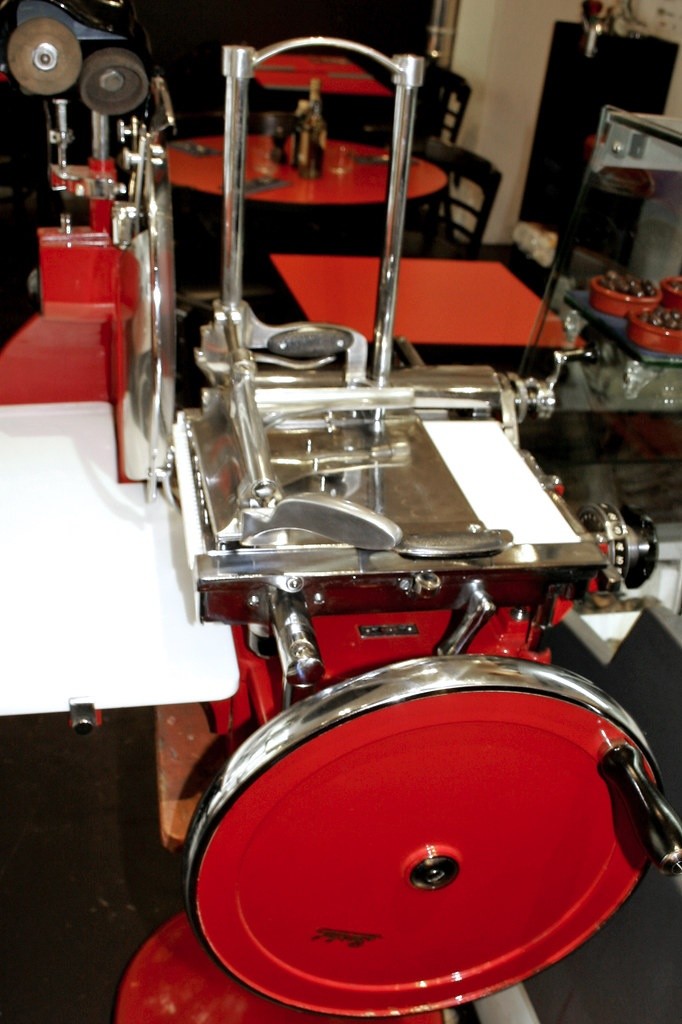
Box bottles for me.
[298,79,328,179]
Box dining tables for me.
[163,137,447,204]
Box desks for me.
[252,53,397,100]
[269,251,586,349]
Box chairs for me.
[375,55,503,260]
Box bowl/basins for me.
[588,274,662,317]
[660,275,682,307]
[624,308,682,354]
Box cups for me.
[261,110,295,166]
[331,142,353,176]
